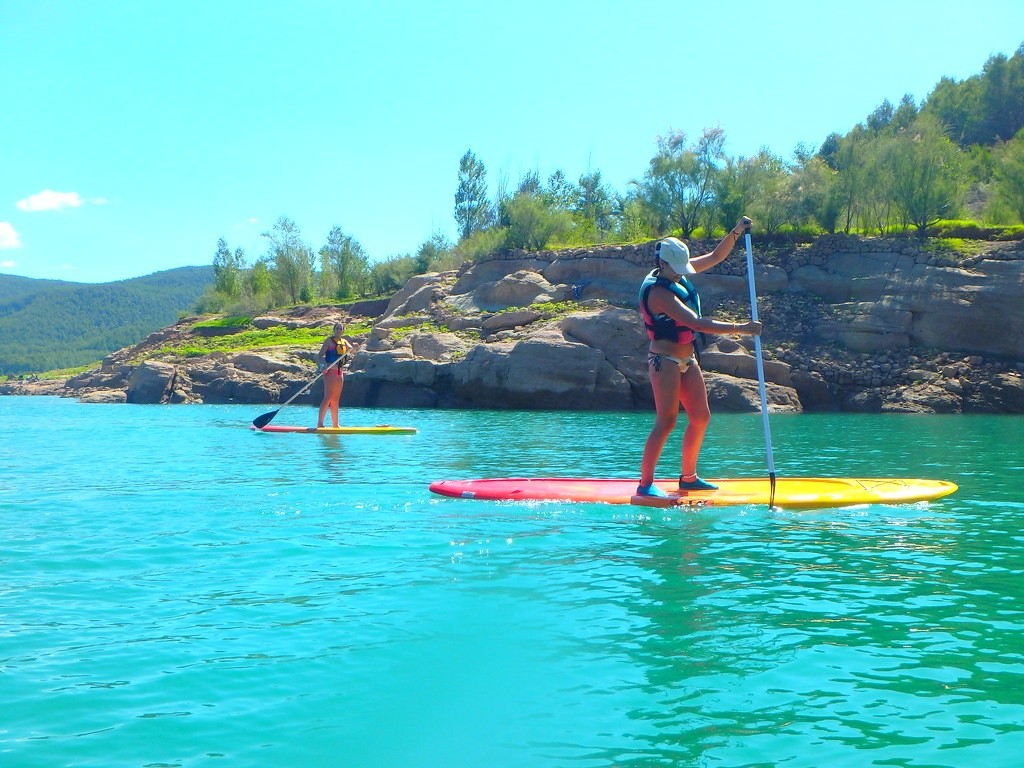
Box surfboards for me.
[249,424,418,436]
[427,472,963,510]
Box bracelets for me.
[733,229,741,241]
[733,322,735,335]
[319,364,324,369]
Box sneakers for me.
[637,482,676,500]
[678,475,718,492]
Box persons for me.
[317,321,359,427]
[636,216,762,498]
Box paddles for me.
[252,336,366,430]
[740,213,778,512]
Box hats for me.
[659,237,697,276]
